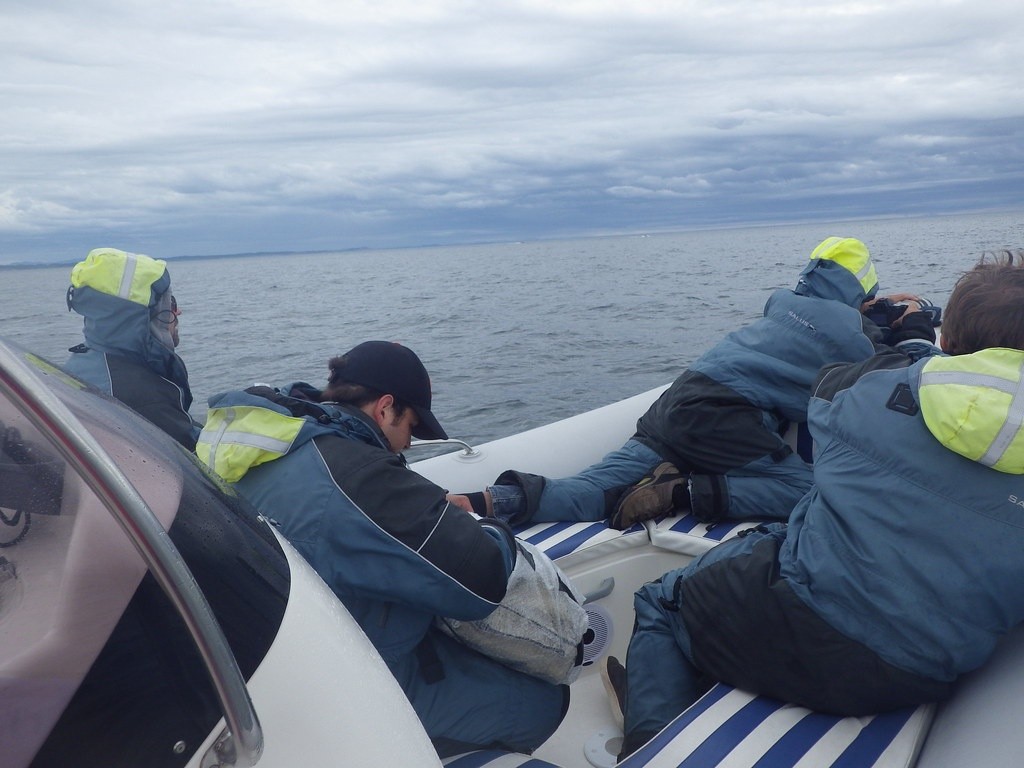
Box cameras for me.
[864,297,909,328]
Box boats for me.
[0,338,1023,766]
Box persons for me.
[195,339,568,756]
[62,248,203,453]
[452,238,936,530]
[606,248,1024,763]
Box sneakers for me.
[601,655,626,733]
[446,492,487,517]
[609,461,689,531]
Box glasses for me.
[171,295,177,312]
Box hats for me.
[328,341,449,440]
